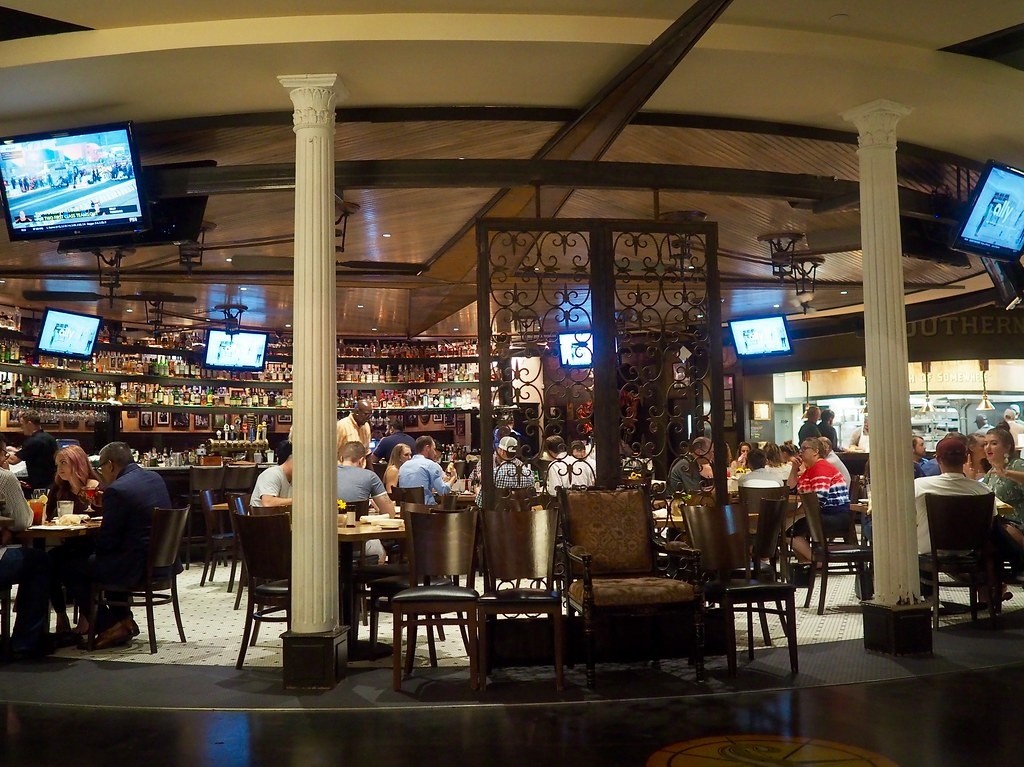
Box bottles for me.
[446,459,454,477]
[0,306,293,408]
[141,446,173,467]
[336,337,498,408]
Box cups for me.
[346,505,356,528]
[254,452,262,462]
[171,452,181,467]
[33,489,47,500]
[30,498,42,526]
[57,500,73,517]
[265,450,274,463]
[452,479,472,491]
[623,457,652,470]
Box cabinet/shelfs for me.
[0,326,518,416]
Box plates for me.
[858,499,868,503]
[381,526,399,528]
[460,493,474,495]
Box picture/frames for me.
[127,410,138,418]
[433,414,443,422]
[259,414,275,431]
[6,411,61,426]
[194,414,210,429]
[443,413,456,427]
[85,422,95,428]
[171,412,190,428]
[139,411,154,428]
[455,413,465,436]
[405,415,418,427]
[420,414,430,424]
[212,414,225,428]
[156,411,170,425]
[62,421,79,427]
[397,415,405,429]
[277,414,293,424]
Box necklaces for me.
[76,487,83,496]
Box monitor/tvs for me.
[727,313,794,358]
[34,306,103,361]
[203,328,269,372]
[0,120,217,254]
[950,157,1024,262]
[557,329,622,370]
[897,185,972,270]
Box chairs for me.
[0,485,1007,696]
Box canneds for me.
[423,394,429,410]
[346,505,356,528]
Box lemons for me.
[39,495,48,503]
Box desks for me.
[849,503,1014,614]
[290,522,406,661]
[654,513,758,578]
[12,522,101,650]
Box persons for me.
[739,448,784,581]
[618,437,634,457]
[912,404,1024,479]
[336,400,597,508]
[666,436,719,567]
[77,441,184,650]
[0,433,48,664]
[978,421,1024,536]
[338,441,396,661]
[249,441,292,507]
[16,210,31,224]
[729,437,851,496]
[974,196,995,236]
[46,444,108,635]
[11,152,135,193]
[7,413,59,523]
[785,437,851,573]
[914,438,998,603]
[797,406,870,453]
[93,205,104,215]
[780,329,787,347]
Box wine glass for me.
[340,410,390,427]
[1,398,110,422]
[83,487,96,512]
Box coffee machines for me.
[441,442,467,461]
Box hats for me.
[498,436,518,452]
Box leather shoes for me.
[77,616,139,651]
[121,618,139,636]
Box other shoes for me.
[71,611,89,635]
[56,613,71,633]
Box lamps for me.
[801,370,811,421]
[859,365,868,415]
[977,359,995,410]
[918,361,938,412]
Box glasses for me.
[95,460,109,475]
[0,447,10,460]
[800,447,816,452]
